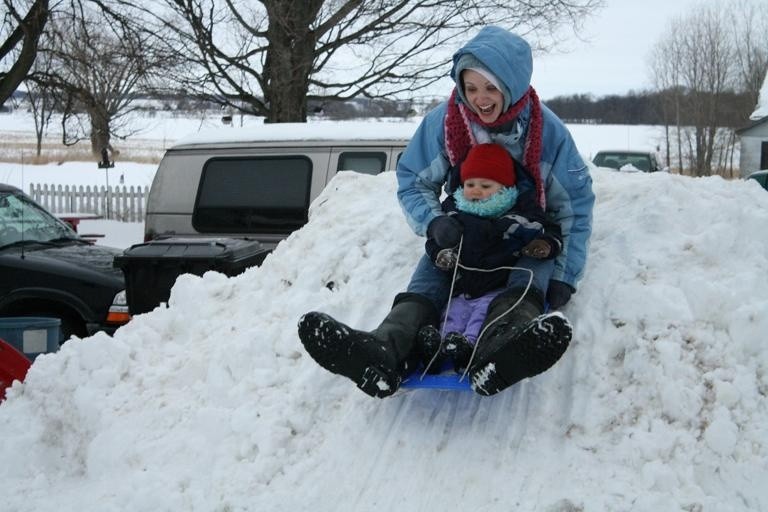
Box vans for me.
[144,122,419,242]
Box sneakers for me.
[417,322,470,375]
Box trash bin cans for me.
[113,238,273,321]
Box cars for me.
[1,183,132,346]
[591,149,663,173]
[744,169,768,191]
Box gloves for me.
[521,238,552,258]
[545,279,572,310]
[426,215,465,249]
[435,248,461,272]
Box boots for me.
[296,294,438,400]
[468,284,572,397]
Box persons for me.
[416,142,565,376]
[297,25,596,400]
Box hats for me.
[455,54,511,114]
[460,142,515,188]
